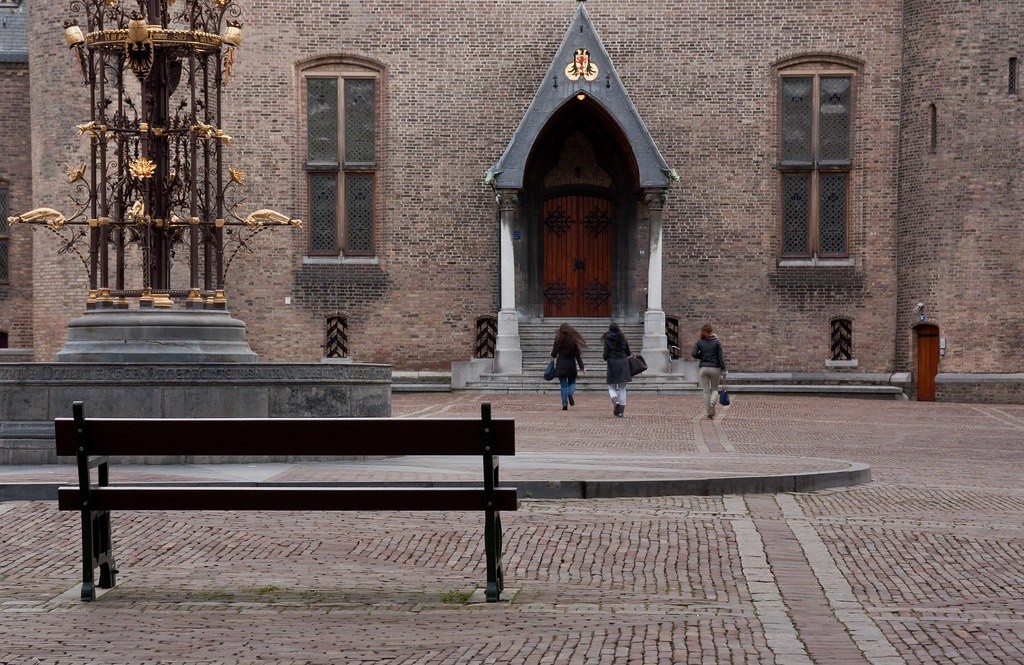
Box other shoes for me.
[563,405,567,409]
[710,404,714,416]
[707,415,713,420]
[568,395,574,405]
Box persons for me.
[603,323,631,417]
[549,323,585,410]
[692,325,725,419]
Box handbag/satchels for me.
[720,376,730,405]
[544,356,555,381]
[626,352,648,376]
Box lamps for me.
[913,303,925,320]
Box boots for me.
[612,397,620,416]
[619,405,624,417]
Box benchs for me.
[54,401,524,603]
